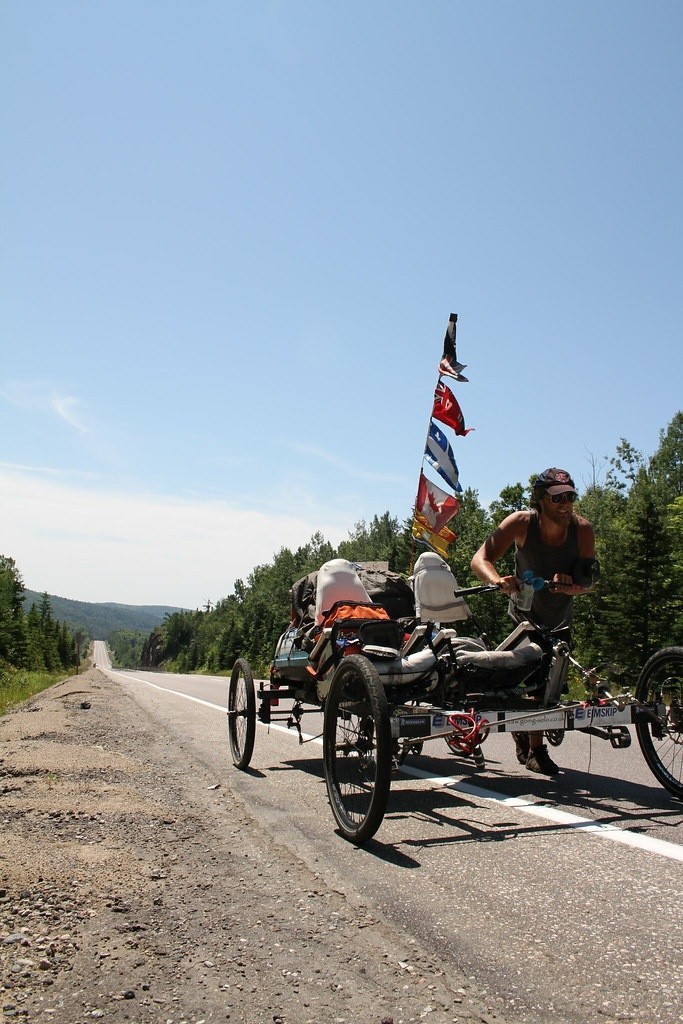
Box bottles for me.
[516,579,535,611]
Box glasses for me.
[549,491,577,505]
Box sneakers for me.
[525,743,559,775]
[511,731,529,764]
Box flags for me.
[424,422,464,493]
[412,510,456,559]
[415,474,461,534]
[437,320,468,382]
[431,381,475,437]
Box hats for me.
[532,467,575,496]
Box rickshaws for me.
[224,552,682,846]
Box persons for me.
[470,468,595,775]
